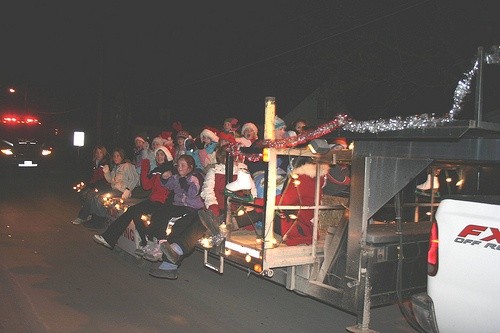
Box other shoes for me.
[93,235,110,248]
[148,267,177,278]
[72,218,85,224]
[161,241,179,264]
[199,210,217,235]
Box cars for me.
[0,110,55,175]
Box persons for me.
[275,116,351,246]
[72,117,266,279]
[415,161,465,192]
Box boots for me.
[225,170,251,191]
[85,214,105,230]
[142,237,167,263]
[134,235,159,257]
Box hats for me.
[135,136,145,144]
[155,145,175,162]
[153,136,168,146]
[176,130,189,139]
[200,127,219,143]
[241,122,257,137]
[275,115,286,130]
[224,117,239,131]
